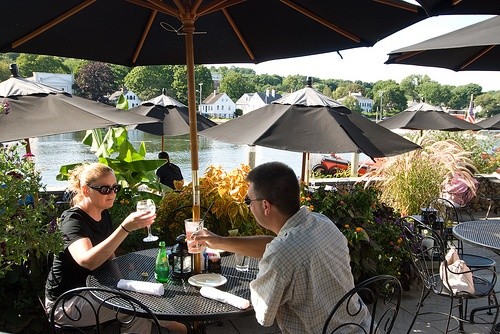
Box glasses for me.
[243,196,265,207]
[89,184,122,196]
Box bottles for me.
[188,273,229,287]
[155,241,170,283]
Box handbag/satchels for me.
[439,248,476,295]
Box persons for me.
[42,163,186,334]
[156,152,184,190]
[184,161,380,334]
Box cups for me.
[184,218,206,254]
[234,253,251,271]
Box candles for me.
[422,239,434,256]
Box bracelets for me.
[120,224,130,233]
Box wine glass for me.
[136,199,159,242]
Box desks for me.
[85,245,261,334]
[475,175,500,216]
[452,220,500,334]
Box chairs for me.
[25,174,500,334]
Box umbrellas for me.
[383,16,500,72]
[120,85,218,152]
[472,112,500,132]
[197,76,423,184]
[416,0,500,16]
[0,0,438,275]
[0,62,164,210]
[372,101,482,138]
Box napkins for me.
[199,286,250,310]
[117,279,164,296]
